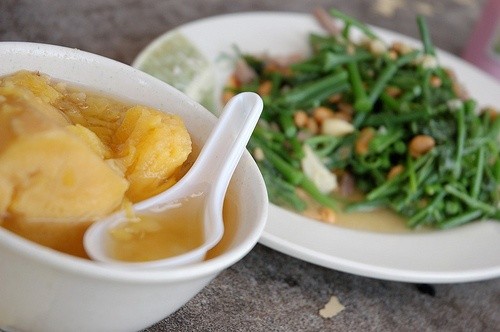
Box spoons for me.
[82,91,265,269]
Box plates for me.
[131,10,500,282]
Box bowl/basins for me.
[0,41,269,332]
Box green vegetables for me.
[208,9,500,230]
